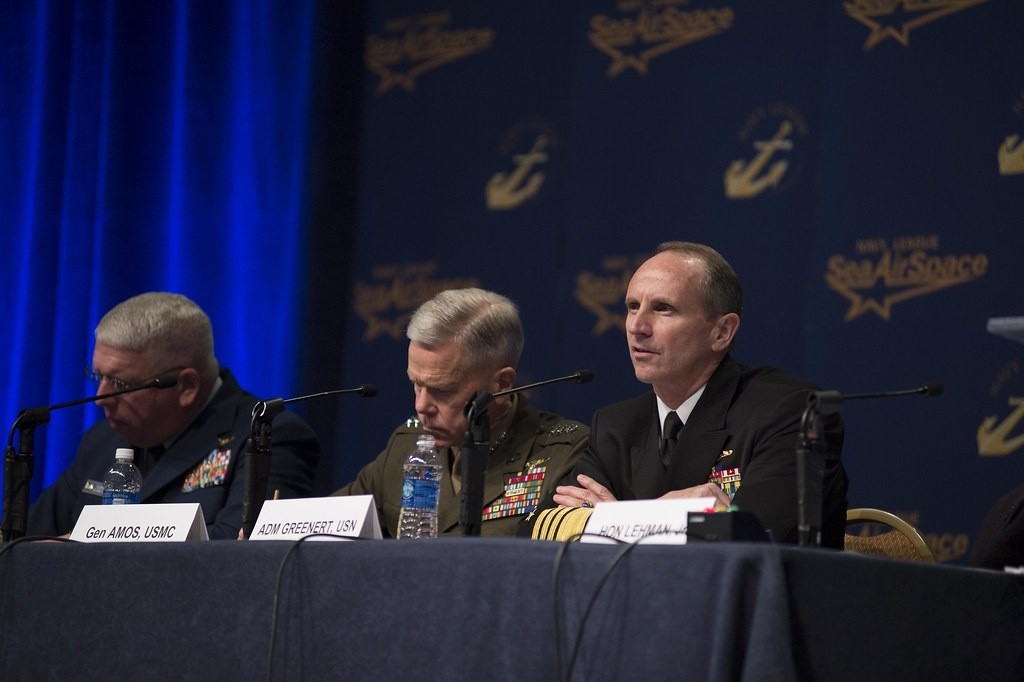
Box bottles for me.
[396,435,443,540]
[102,448,143,505]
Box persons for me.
[29,291,324,539]
[552,240,847,549]
[330,288,608,538]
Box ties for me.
[658,410,683,475]
[141,443,167,478]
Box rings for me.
[581,501,594,509]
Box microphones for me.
[797,380,946,549]
[459,368,594,538]
[243,382,377,540]
[0,375,179,543]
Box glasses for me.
[82,362,185,399]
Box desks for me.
[1,540,1024,681]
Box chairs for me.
[845,507,935,562]
